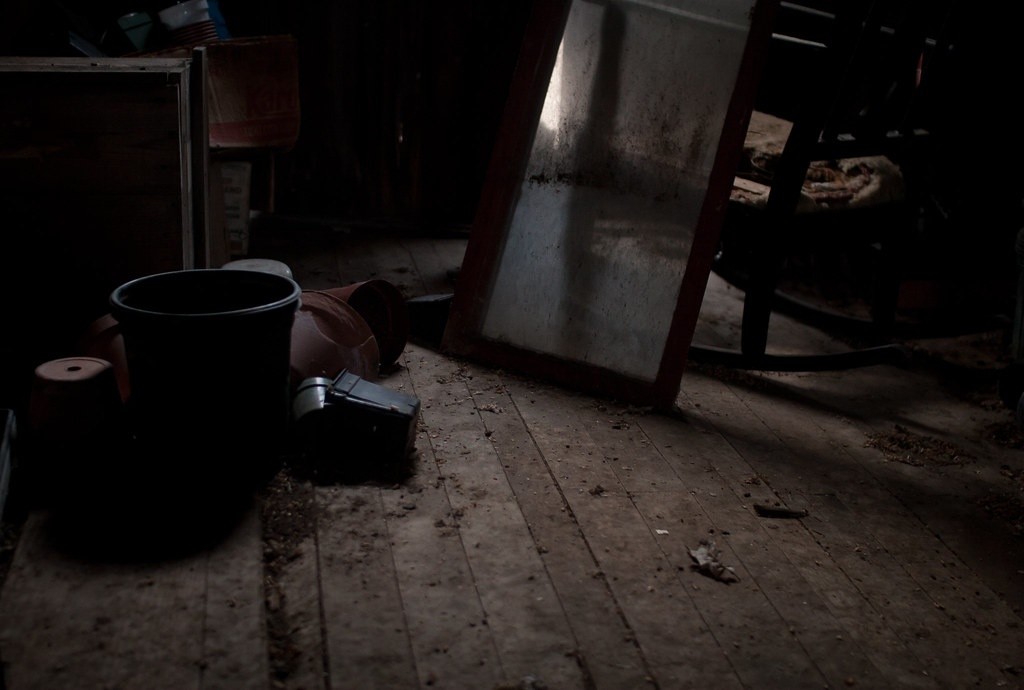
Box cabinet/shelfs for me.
[710,0,927,365]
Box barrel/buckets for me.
[109,266,303,484]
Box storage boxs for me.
[122,36,300,151]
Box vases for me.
[32,256,423,476]
